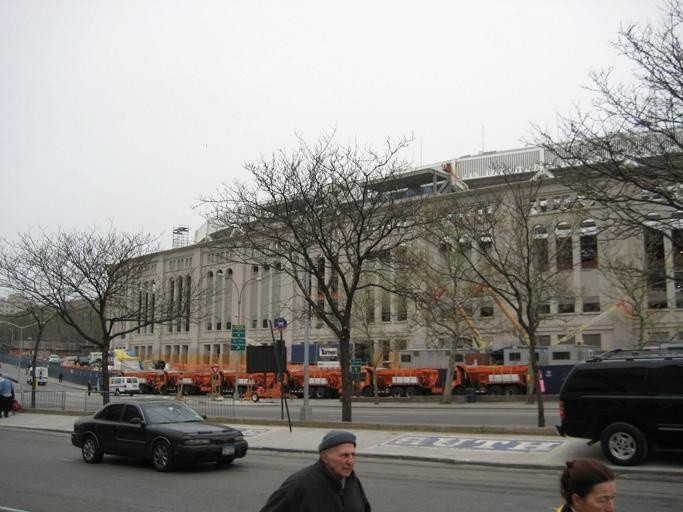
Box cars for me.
[48,355,87,365]
[71,400,248,472]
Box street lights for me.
[0,321,47,382]
[217,272,265,401]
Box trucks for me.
[373,366,466,396]
[27,367,49,386]
[123,371,292,402]
[457,365,546,394]
[287,365,374,398]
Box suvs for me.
[554,348,683,466]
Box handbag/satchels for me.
[11,401,20,411]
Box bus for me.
[88,352,114,364]
[97,376,140,396]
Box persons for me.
[59,371,63,382]
[0,373,16,417]
[556,460,617,512]
[115,389,120,396]
[88,380,92,396]
[258,430,371,512]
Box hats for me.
[319,431,356,452]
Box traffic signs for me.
[231,325,246,352]
[351,359,362,375]
